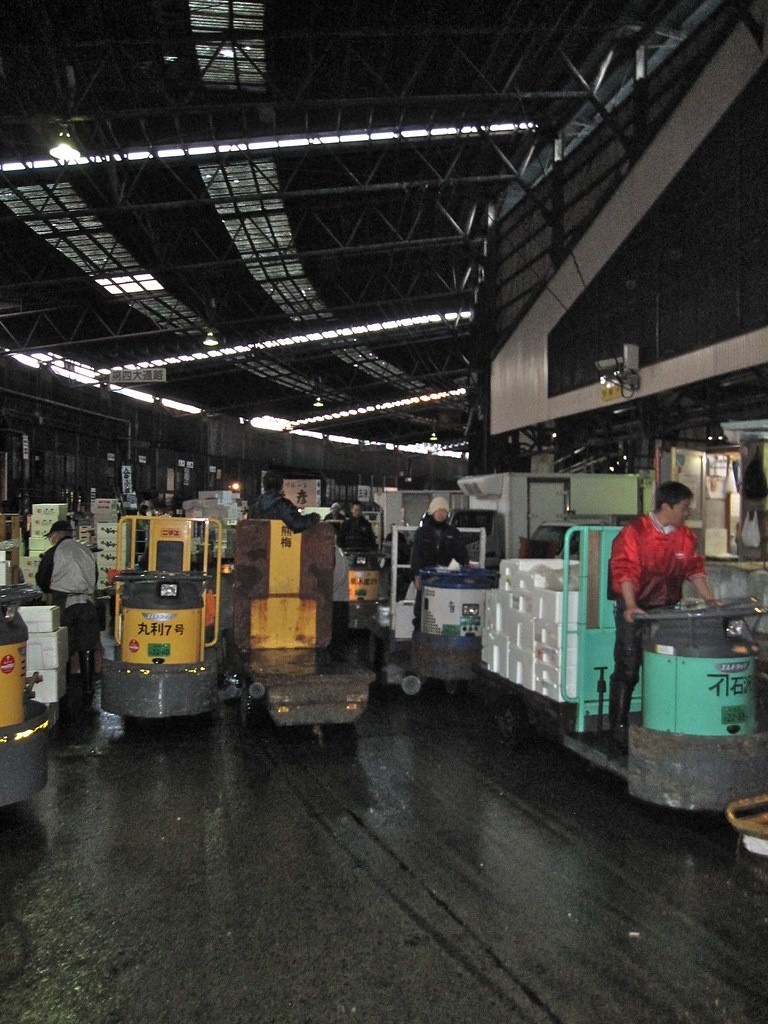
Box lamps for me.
[49,126,82,161]
[203,331,219,347]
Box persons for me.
[410,497,476,646]
[385,523,405,541]
[35,520,98,695]
[20,508,32,556]
[324,503,346,520]
[248,471,320,533]
[138,506,149,528]
[338,502,376,548]
[155,489,166,508]
[609,482,722,756]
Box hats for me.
[428,496,449,515]
[330,502,342,510]
[43,520,73,536]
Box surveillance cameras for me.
[596,357,624,373]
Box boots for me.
[608,679,633,744]
[68,652,81,674]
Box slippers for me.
[90,649,102,673]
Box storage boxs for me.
[681,562,767,632]
[79,499,131,589]
[375,604,391,628]
[17,606,69,730]
[182,491,243,559]
[481,558,580,703]
[0,503,69,595]
